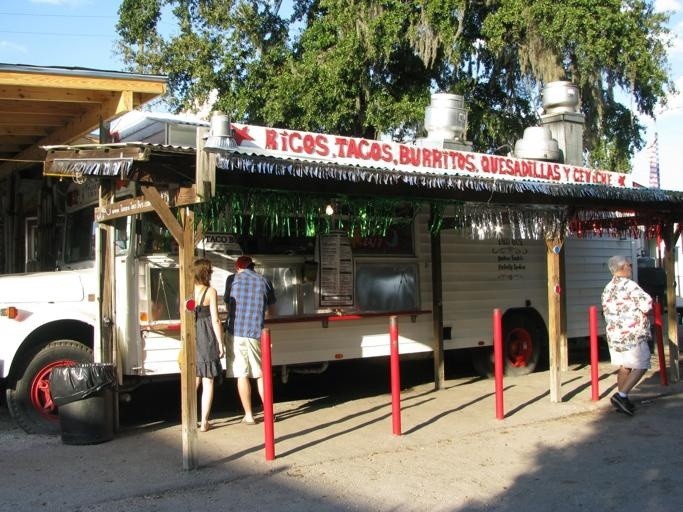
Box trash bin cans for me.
[639,266,666,317]
[52,362,117,445]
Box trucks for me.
[0,104,644,437]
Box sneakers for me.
[610,393,636,416]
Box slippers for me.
[242,416,256,426]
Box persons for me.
[175,258,225,432]
[222,255,277,424]
[600,256,654,416]
